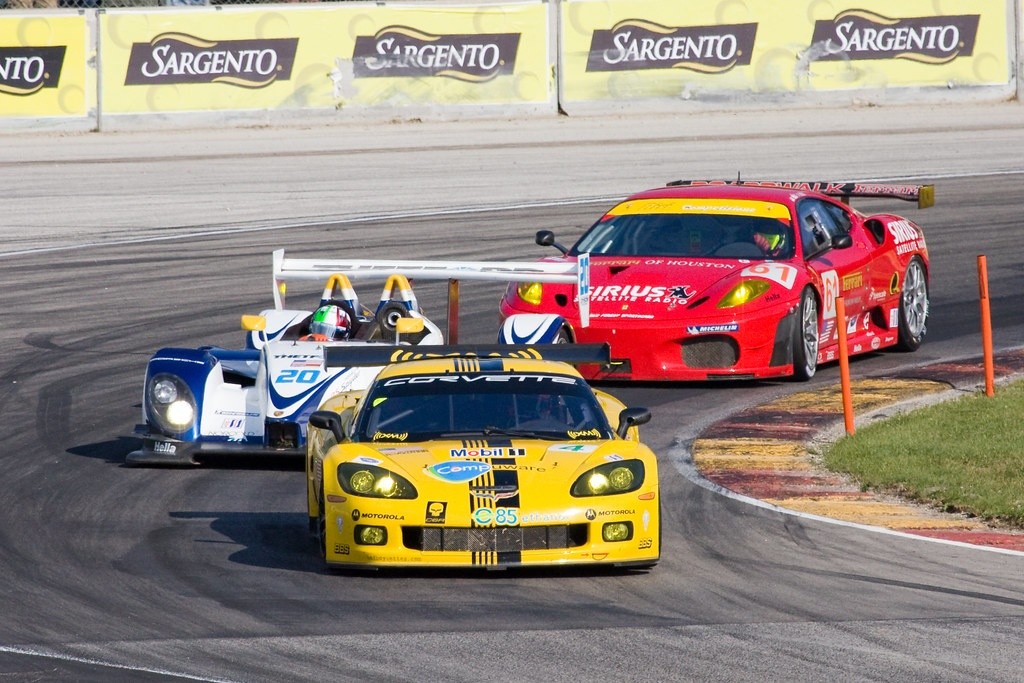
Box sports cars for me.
[125,250,590,465]
[497,179,936,382]
[305,339,662,572]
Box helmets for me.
[508,392,553,421]
[309,305,352,342]
[755,222,788,252]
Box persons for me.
[308,305,351,340]
[503,379,575,426]
[748,218,789,258]
